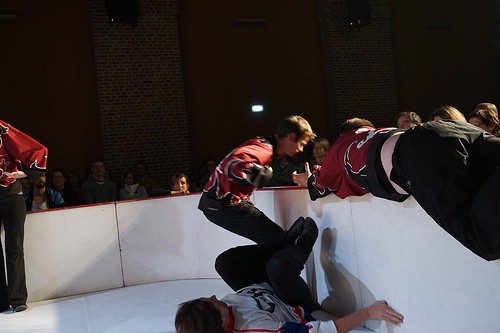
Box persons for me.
[198,115,318,245]
[0,120,48,312]
[20,102,500,211]
[290,118,500,261]
[174,215,404,333]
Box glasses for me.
[312,146,323,151]
[52,176,62,180]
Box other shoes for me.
[15,305,26,312]
[297,217,318,258]
[284,217,303,245]
